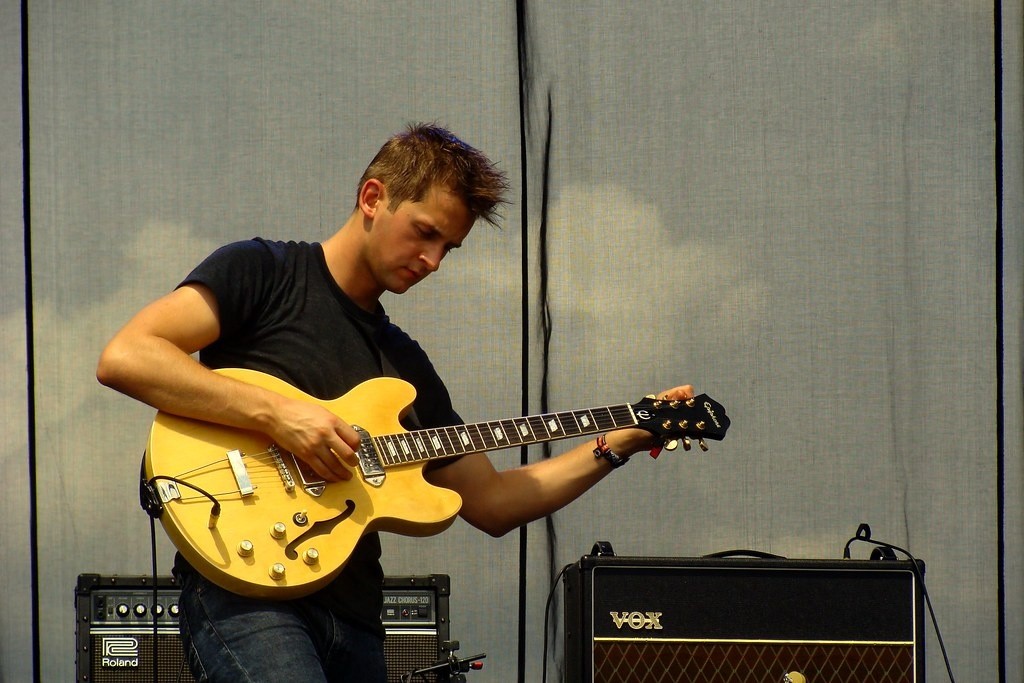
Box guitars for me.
[143,365,731,601]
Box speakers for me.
[74,573,451,683]
[563,556,927,683]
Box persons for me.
[97,126,693,683]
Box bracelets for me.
[593,434,631,468]
